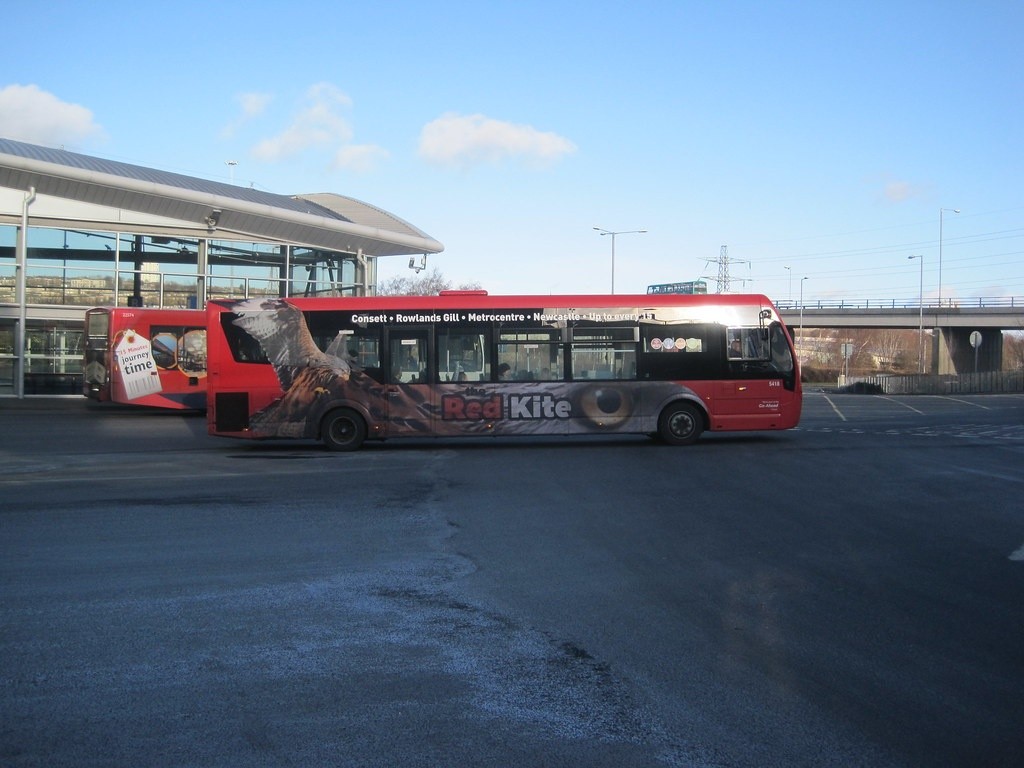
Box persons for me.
[458,373,467,381]
[349,349,359,361]
[391,366,402,380]
[498,363,513,380]
[728,341,741,357]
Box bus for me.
[81,306,487,410]
[205,294,802,446]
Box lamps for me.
[207,217,216,226]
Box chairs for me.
[419,366,622,380]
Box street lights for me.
[908,255,923,374]
[592,226,647,293]
[938,208,961,307]
[785,266,792,298]
[799,276,810,376]
